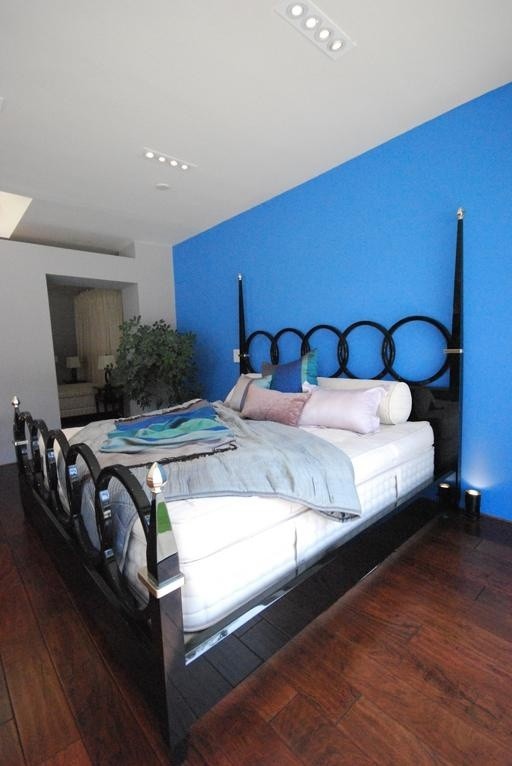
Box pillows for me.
[224,346,412,435]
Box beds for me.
[13,208,461,750]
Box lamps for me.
[96,352,120,390]
[66,356,80,383]
[464,489,483,520]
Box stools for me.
[95,393,123,419]
